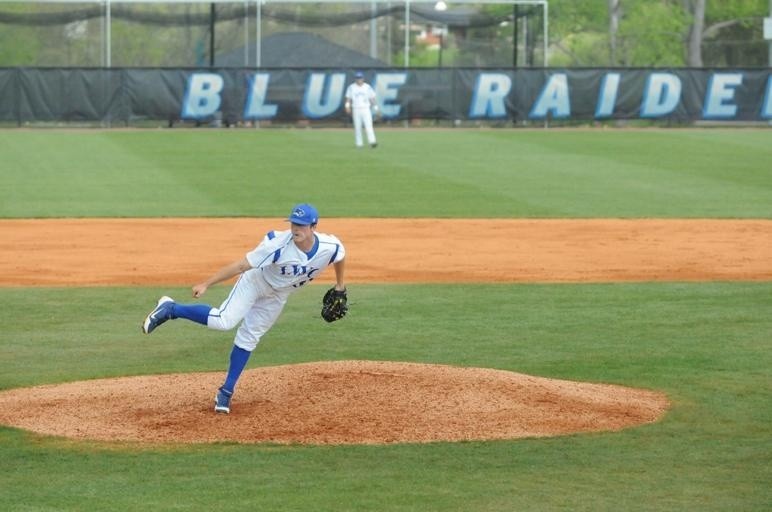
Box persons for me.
[142,204,346,414]
[344,71,380,150]
[415,22,441,52]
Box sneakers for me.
[215,388,232,413]
[143,296,176,334]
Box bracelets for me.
[374,105,379,111]
[345,103,351,109]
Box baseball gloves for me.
[321,286,348,322]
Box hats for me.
[285,204,318,225]
[355,72,363,79]
